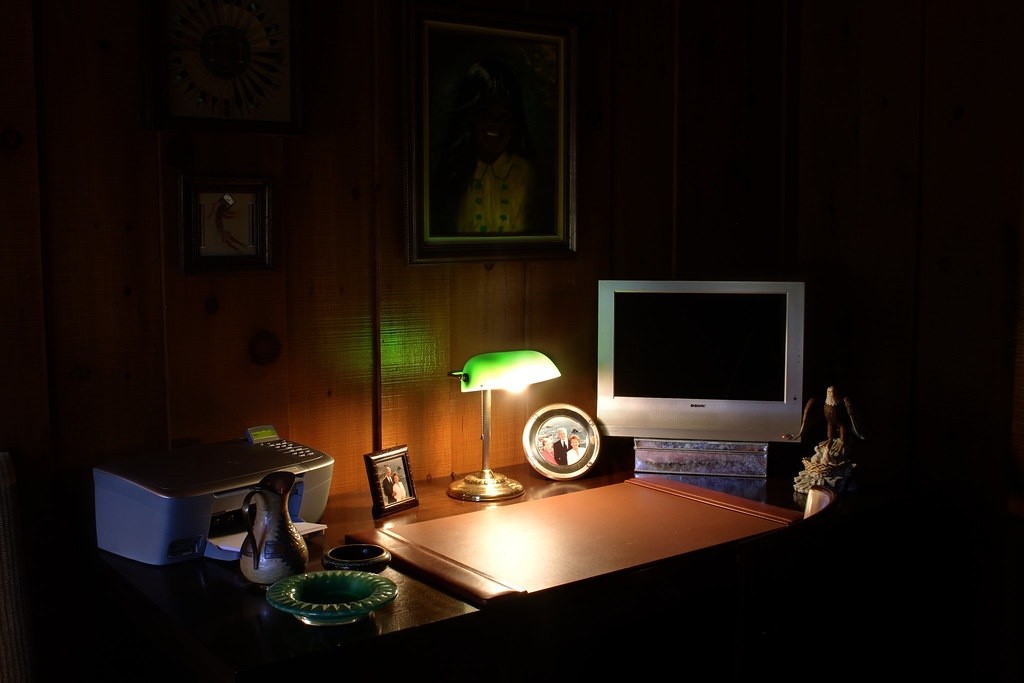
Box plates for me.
[265,571,399,625]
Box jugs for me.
[240,471,308,589]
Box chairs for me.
[480,485,835,683]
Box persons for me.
[382,467,406,505]
[553,427,585,466]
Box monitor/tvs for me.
[596,281,805,443]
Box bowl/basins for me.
[321,544,392,573]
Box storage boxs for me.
[634,438,770,479]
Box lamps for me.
[447,351,564,502]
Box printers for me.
[92,424,334,566]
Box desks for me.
[0,453,885,683]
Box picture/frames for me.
[177,174,274,274]
[400,8,579,266]
[363,442,419,516]
[521,402,600,480]
[144,0,304,135]
